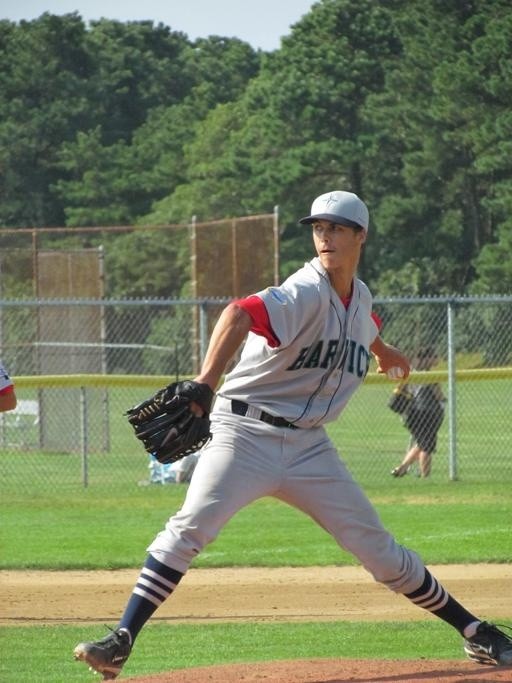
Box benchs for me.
[0,398,41,449]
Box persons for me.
[0,361,17,412]
[390,347,446,477]
[72,189,512,679]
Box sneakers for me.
[464,622,512,667]
[75,631,131,678]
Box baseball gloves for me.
[127,380,212,464]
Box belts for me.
[231,399,297,431]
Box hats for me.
[300,191,370,232]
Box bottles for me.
[148,454,173,481]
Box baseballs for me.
[386,366,404,381]
